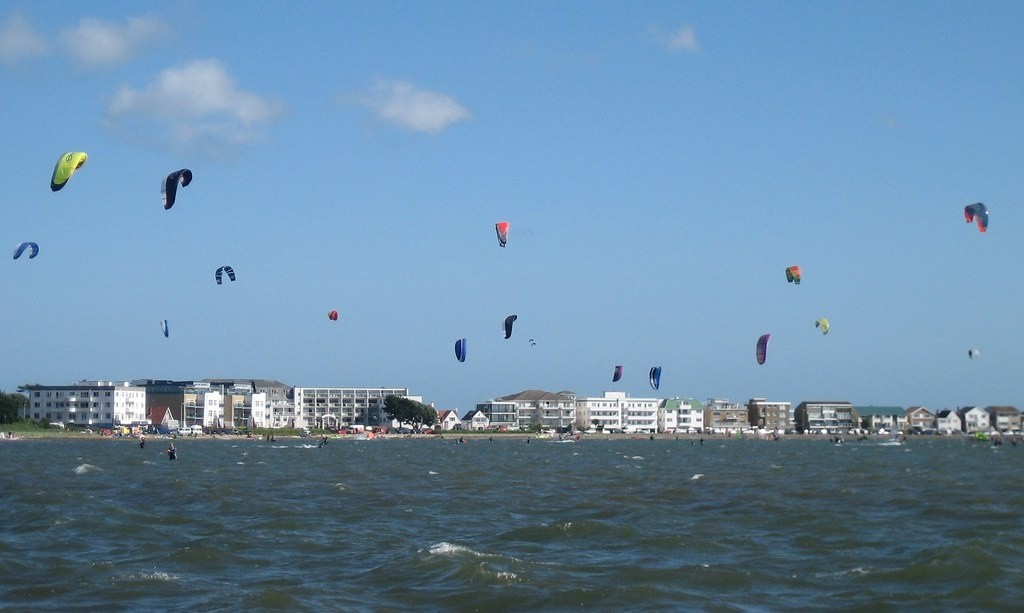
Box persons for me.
[454,439,458,445]
[700,438,704,446]
[489,437,494,442]
[318,435,328,447]
[991,436,1024,448]
[139,438,145,448]
[168,443,177,460]
[526,437,531,446]
[830,436,842,443]
[459,436,464,443]
